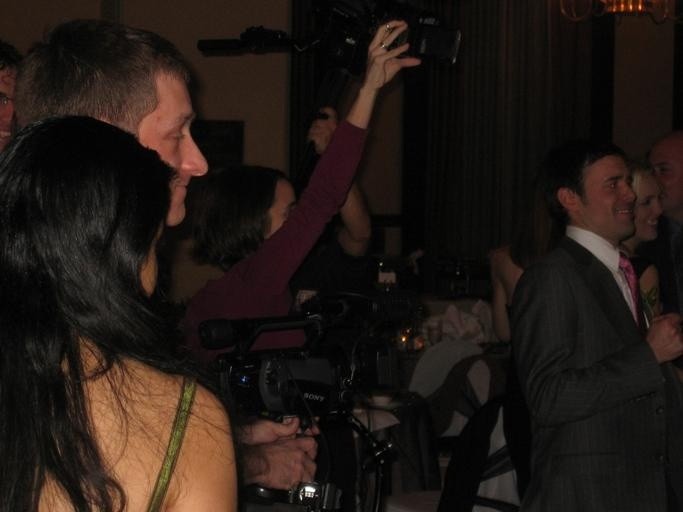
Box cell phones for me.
[388,27,462,63]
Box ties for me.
[618,256,641,330]
